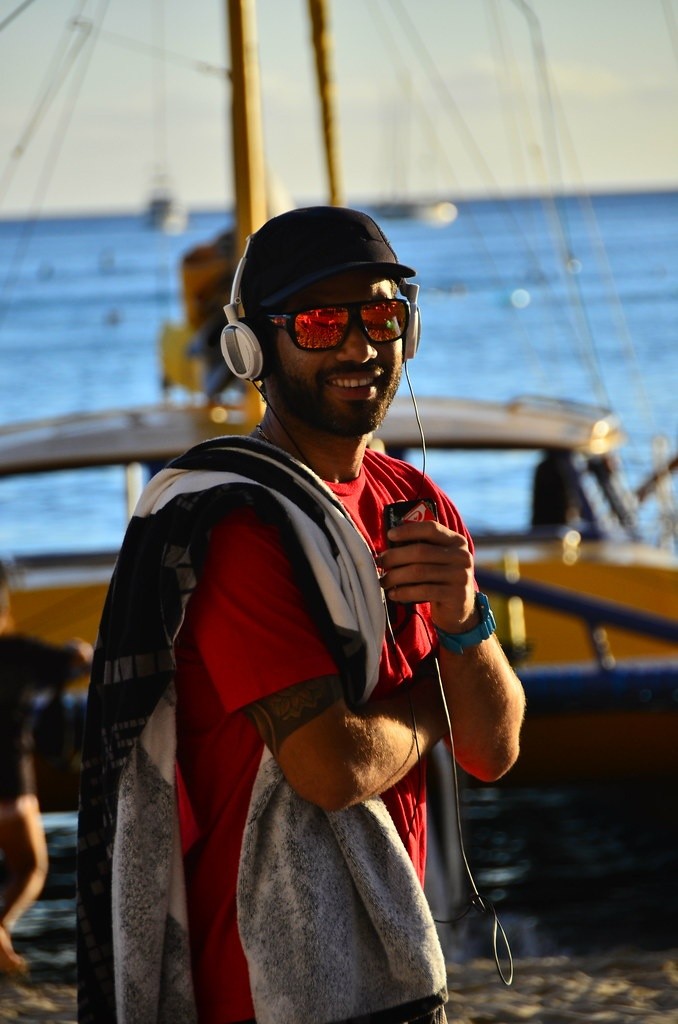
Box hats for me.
[235,206,416,329]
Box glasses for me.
[265,298,411,351]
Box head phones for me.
[220,233,421,382]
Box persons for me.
[0,558,93,973]
[150,203,529,1024]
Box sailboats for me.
[1,0,677,768]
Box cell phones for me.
[381,498,440,606]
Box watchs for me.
[433,589,498,654]
